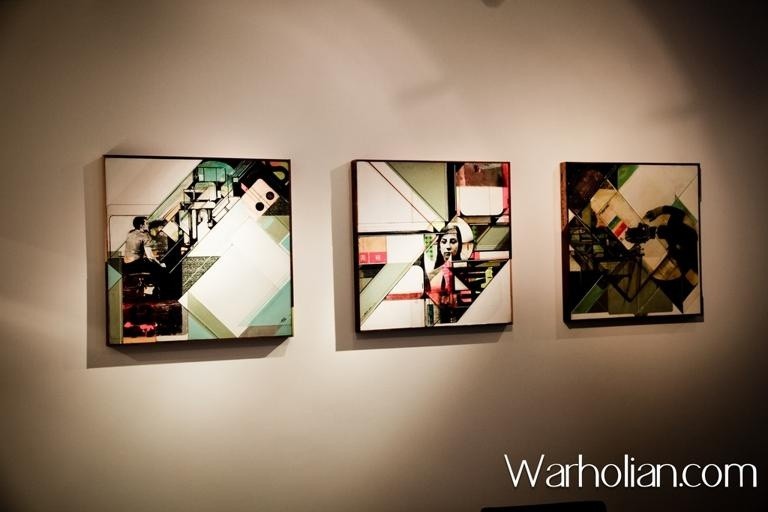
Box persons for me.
[433,225,462,269]
[123,216,168,271]
